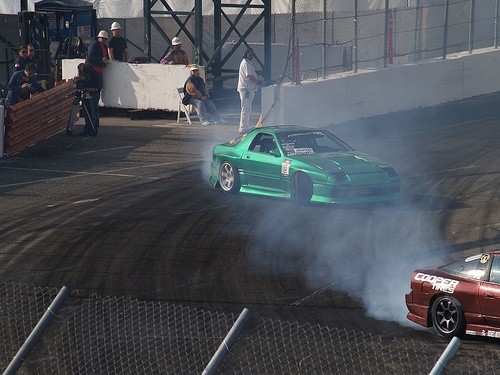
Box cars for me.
[209,126,403,208]
[406,249,500,339]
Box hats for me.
[171,37,182,45]
[97,30,109,39]
[241,47,253,58]
[110,21,122,30]
[190,64,200,70]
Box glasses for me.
[28,49,34,52]
[21,51,28,54]
[99,37,108,40]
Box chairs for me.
[175,86,202,125]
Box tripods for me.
[67,91,97,135]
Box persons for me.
[23,44,35,68]
[5,64,39,106]
[10,46,30,81]
[160,36,188,64]
[107,21,130,63]
[74,62,102,137]
[237,47,265,135]
[183,63,227,125]
[82,30,109,91]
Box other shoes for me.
[202,119,227,125]
